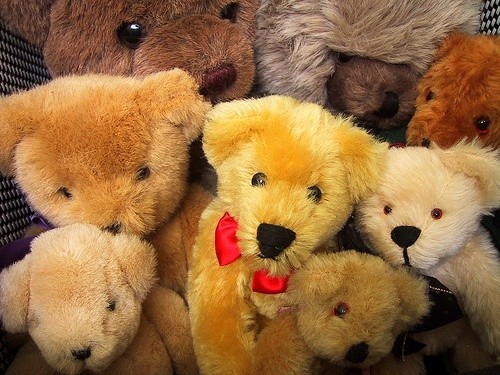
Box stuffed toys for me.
[1,66,213,302]
[1,1,254,102]
[353,135,499,375]
[252,250,433,374]
[188,95,390,375]
[403,31,500,146]
[1,223,200,375]
[256,1,483,130]
